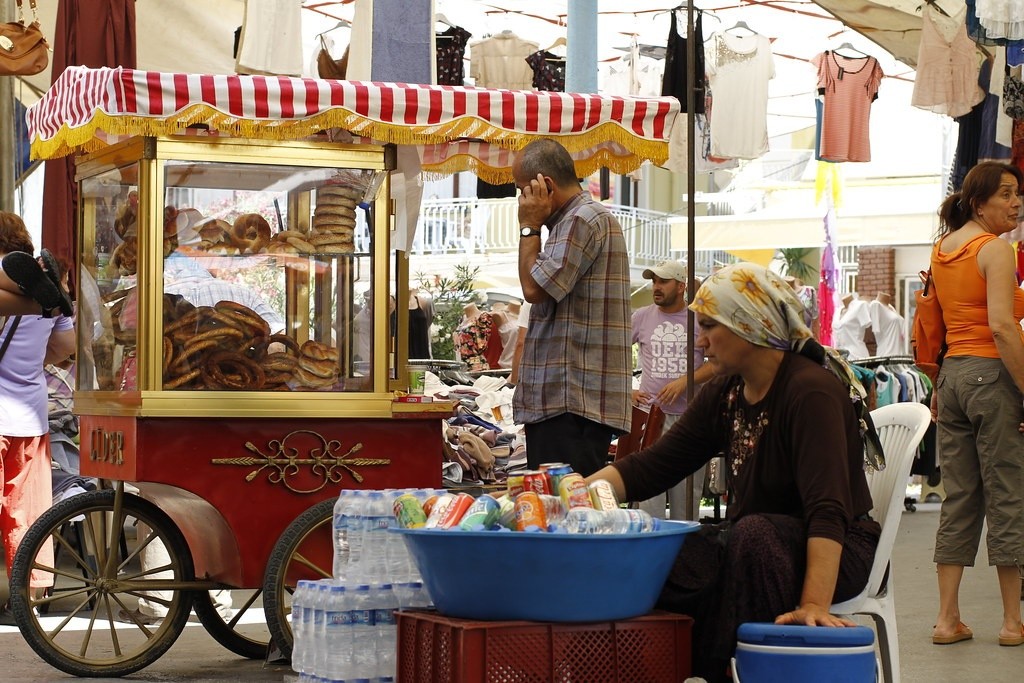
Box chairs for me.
[827,402,932,683]
[615,403,667,508]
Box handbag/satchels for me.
[911,264,946,390]
[0,0,49,75]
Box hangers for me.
[1011,67,1022,83]
[653,0,722,23]
[976,51,982,74]
[435,2,473,38]
[834,43,868,57]
[543,38,567,61]
[315,2,351,40]
[725,21,758,35]
[864,355,920,377]
[427,360,476,385]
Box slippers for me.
[933,621,973,643]
[999,623,1024,645]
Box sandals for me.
[2,251,60,306]
[40,248,74,318]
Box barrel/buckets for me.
[731,623,885,683]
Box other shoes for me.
[0,603,40,624]
[118,608,165,625]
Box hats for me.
[643,260,686,283]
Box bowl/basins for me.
[387,519,702,622]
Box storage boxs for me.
[392,608,694,683]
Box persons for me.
[583,262,890,683]
[511,138,632,478]
[631,259,715,521]
[0,209,78,624]
[511,300,532,384]
[390,283,435,359]
[829,290,905,360]
[929,161,1024,646]
[453,300,523,372]
[167,256,284,334]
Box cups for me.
[406,364,427,396]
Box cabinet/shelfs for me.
[74,133,396,394]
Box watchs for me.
[519,227,540,237]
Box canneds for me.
[393,462,620,533]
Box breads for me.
[92,286,340,392]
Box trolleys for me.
[6,62,683,683]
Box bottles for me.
[292,578,435,682]
[97,253,113,287]
[548,494,660,534]
[332,488,448,585]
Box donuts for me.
[108,184,357,276]
